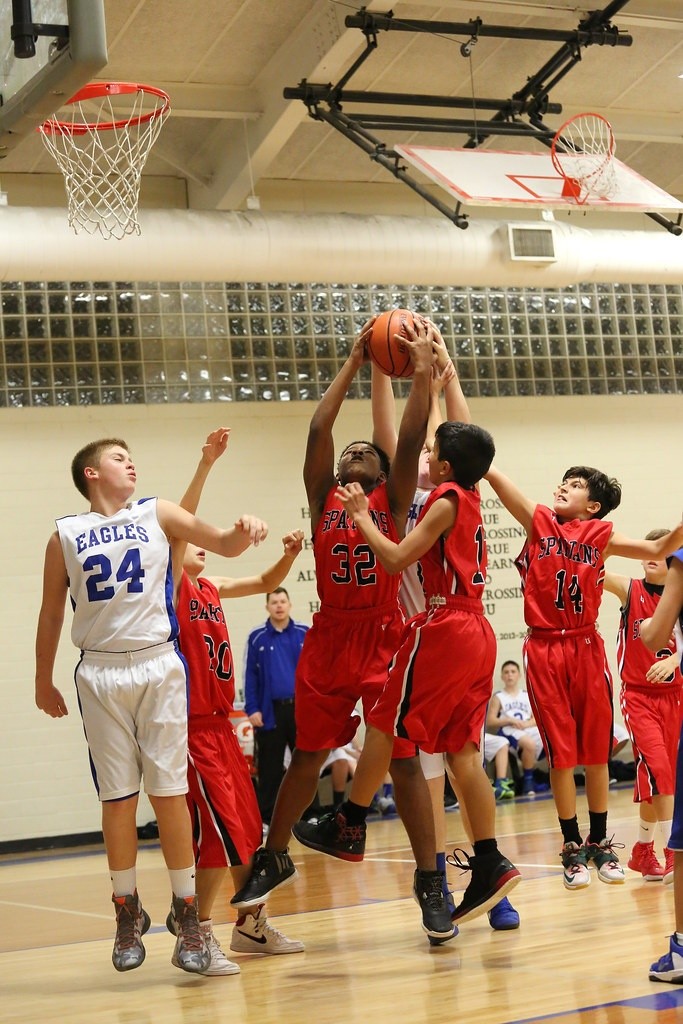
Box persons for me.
[34,438,266,972]
[230,317,521,946]
[171,427,305,954]
[601,528,683,884]
[486,660,617,796]
[281,703,516,814]
[487,466,683,889]
[639,544,683,985]
[243,587,311,834]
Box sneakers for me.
[494,778,514,797]
[111,886,151,972]
[290,807,367,862]
[489,780,505,800]
[584,832,625,883]
[446,848,521,925]
[558,838,590,889]
[648,936,683,983]
[426,879,459,945]
[171,923,241,976]
[230,903,305,953]
[228,847,298,909]
[169,891,211,973]
[411,869,455,937]
[627,840,665,880]
[487,896,518,930]
[662,848,674,885]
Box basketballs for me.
[366,308,435,378]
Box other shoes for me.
[443,797,458,810]
[377,797,393,814]
[524,778,536,796]
